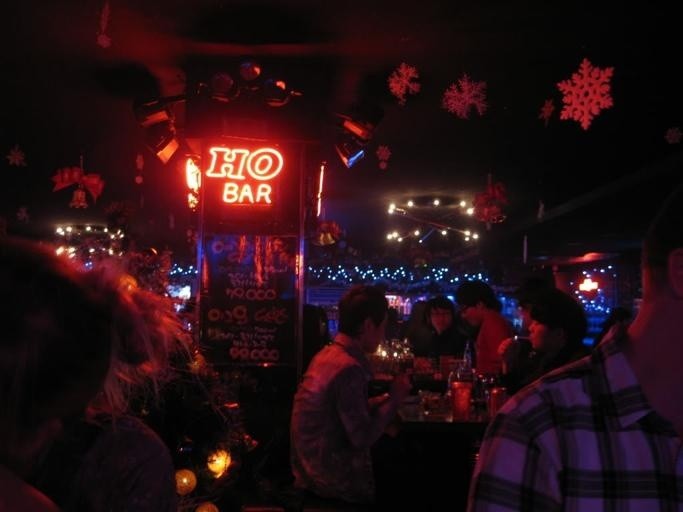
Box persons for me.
[466,185,682,512]
[1,234,192,512]
[288,279,682,512]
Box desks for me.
[397,394,487,423]
[368,366,450,383]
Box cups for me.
[407,341,501,426]
